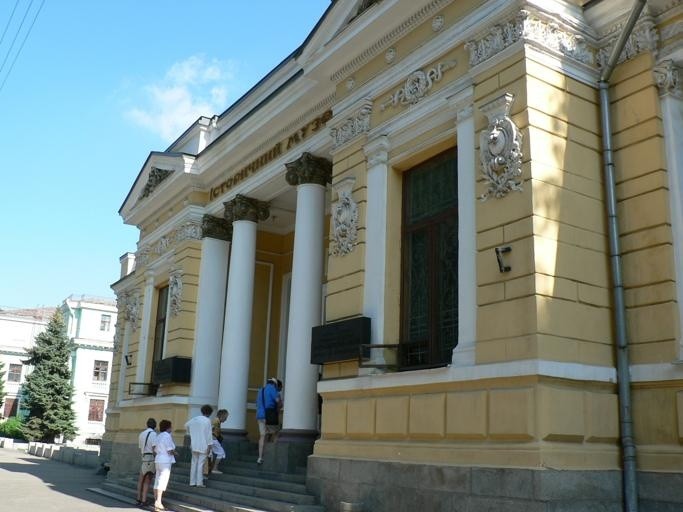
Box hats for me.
[267,377,277,384]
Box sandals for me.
[136,498,168,511]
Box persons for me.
[206,409,229,474]
[256,377,283,465]
[184,404,212,488]
[154,420,177,509]
[137,418,157,507]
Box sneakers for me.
[189,483,208,489]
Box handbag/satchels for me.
[212,432,223,443]
[141,460,157,476]
[265,407,280,426]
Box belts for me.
[143,452,153,456]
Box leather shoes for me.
[211,469,223,474]
